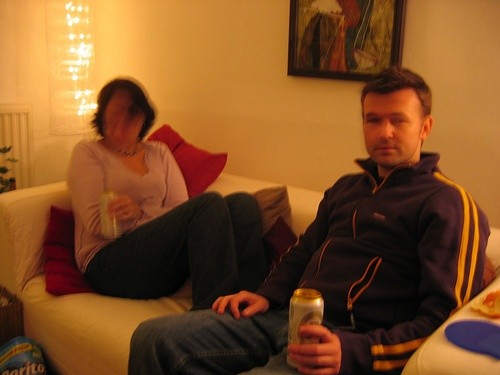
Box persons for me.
[66,78,269,312]
[129,68,491,374]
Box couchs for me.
[0,174,500,375]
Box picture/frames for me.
[287,0,408,79]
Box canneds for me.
[99,191,122,238]
[286,289,325,367]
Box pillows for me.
[255,186,298,269]
[147,124,227,197]
[45,205,92,298]
